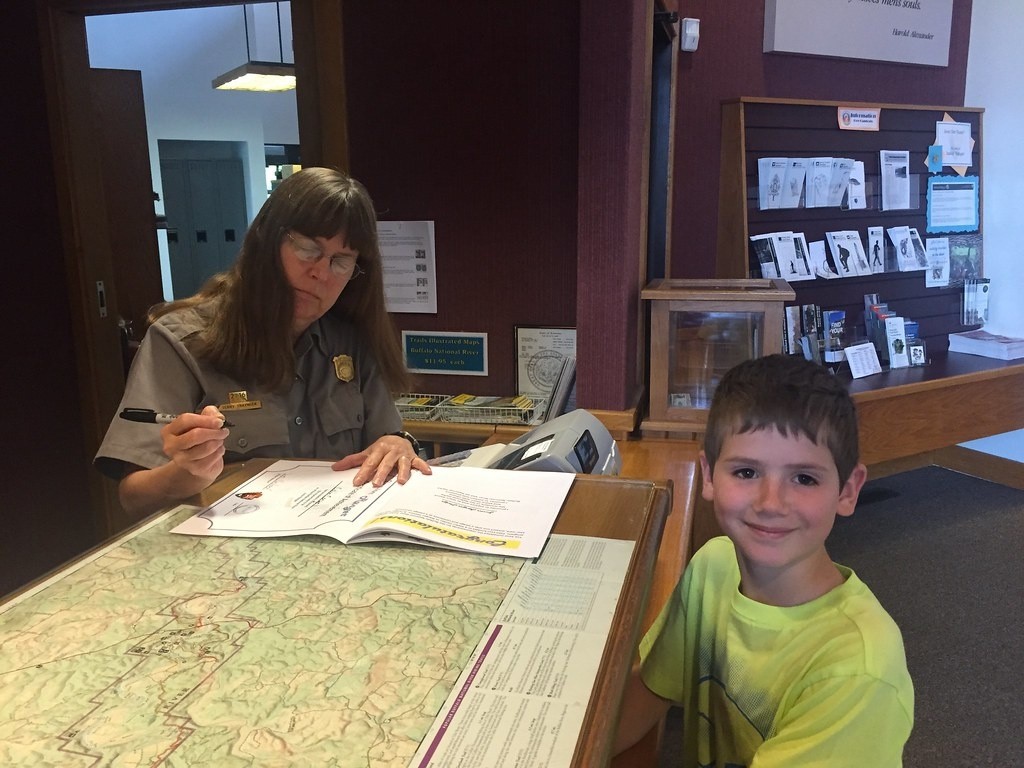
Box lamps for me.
[212,1,296,92]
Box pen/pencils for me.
[119,407,236,429]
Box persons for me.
[93,167,432,520]
[610,353,915,768]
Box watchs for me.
[385,430,420,454]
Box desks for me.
[0,457,674,768]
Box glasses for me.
[281,227,367,281]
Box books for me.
[170,459,576,544]
